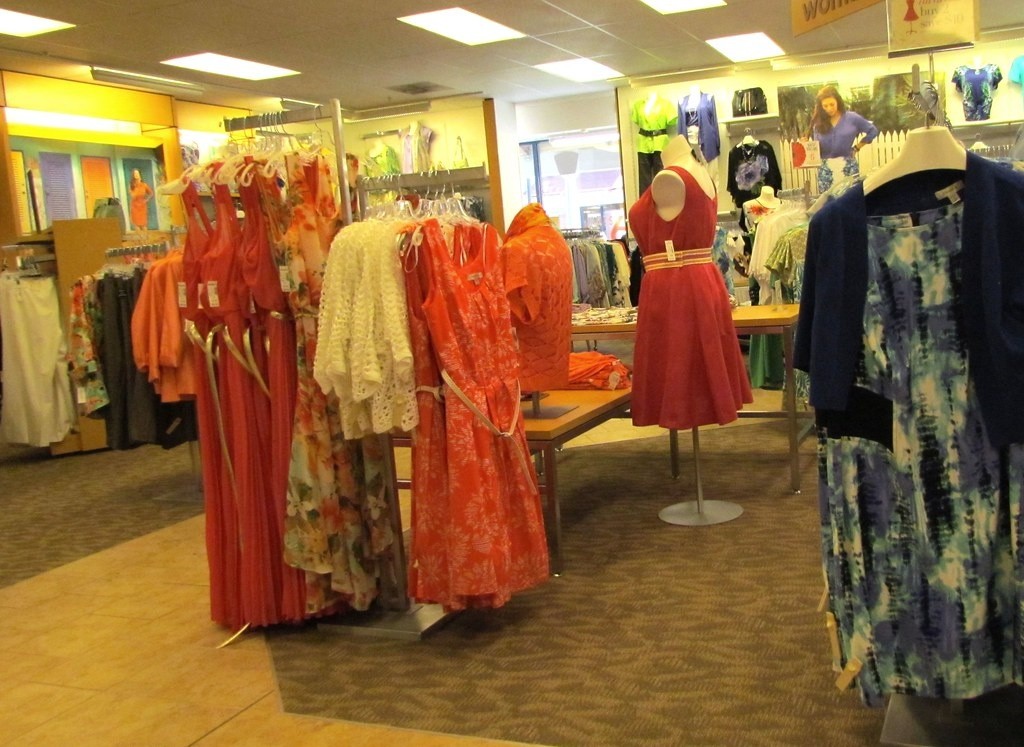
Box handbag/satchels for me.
[732,88,768,117]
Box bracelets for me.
[852,145,860,152]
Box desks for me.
[571,303,815,495]
[391,387,680,578]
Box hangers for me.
[0,245,52,284]
[736,127,759,148]
[969,133,990,152]
[360,167,482,257]
[863,80,966,196]
[564,229,609,247]
[93,242,164,281]
[157,102,347,196]
[776,188,815,221]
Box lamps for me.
[280,96,352,120]
[89,65,205,97]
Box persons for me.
[127,168,154,231]
[807,85,878,195]
[497,202,574,392]
[738,185,801,389]
[628,134,753,431]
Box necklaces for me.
[741,139,757,165]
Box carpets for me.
[265,420,890,747]
[0,442,205,590]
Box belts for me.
[639,128,666,136]
